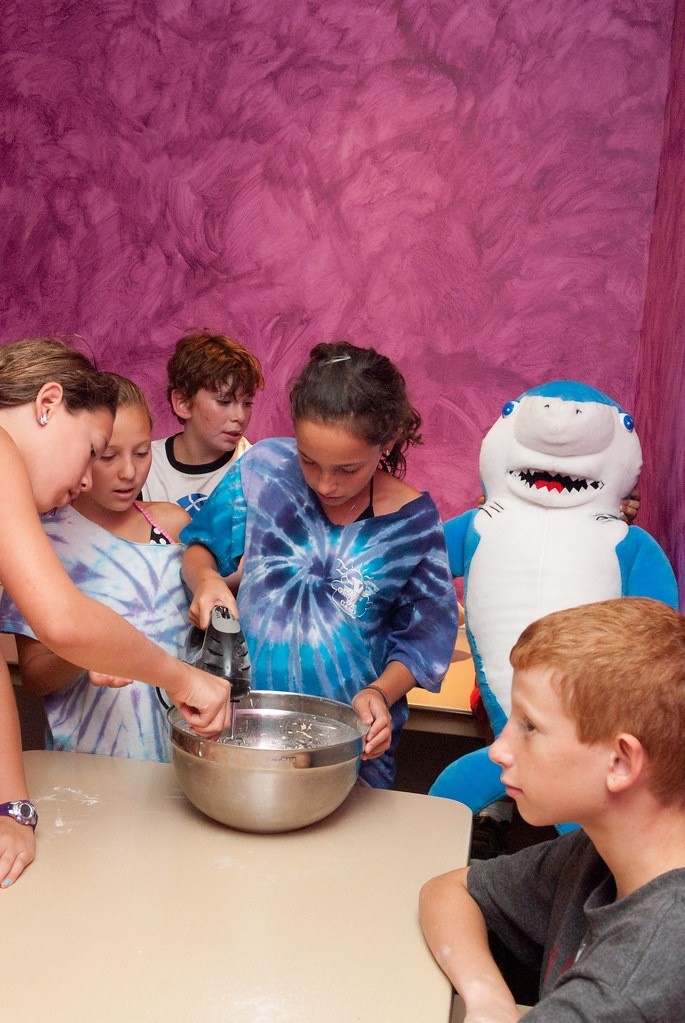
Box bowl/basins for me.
[165,689,371,834]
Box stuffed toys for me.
[427,379,678,836]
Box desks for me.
[0,751,475,1023]
[401,628,484,739]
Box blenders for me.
[182,605,252,746]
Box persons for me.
[419,598,685,1023]
[0,652,36,889]
[0,371,201,763]
[179,340,460,790]
[469,486,641,840]
[135,331,266,520]
[0,325,232,741]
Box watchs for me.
[0,799,38,831]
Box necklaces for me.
[339,490,362,526]
